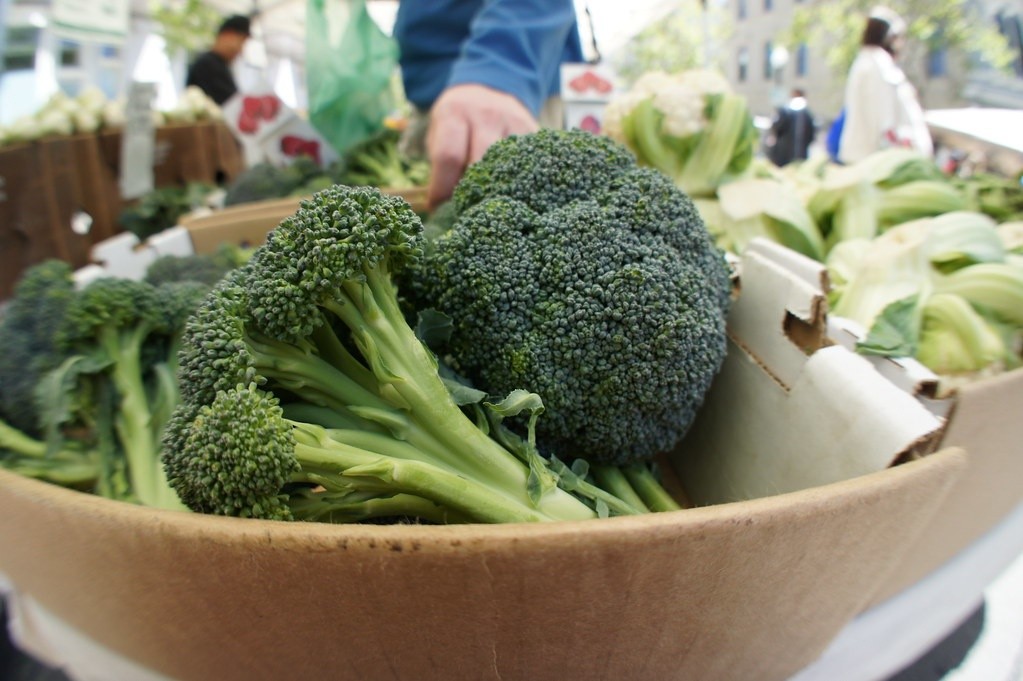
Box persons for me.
[826,107,845,166]
[392,0,601,214]
[768,88,815,166]
[838,8,934,166]
[186,15,251,105]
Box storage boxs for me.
[859,365,1023,624]
[180,185,429,253]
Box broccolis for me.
[0,125,732,527]
